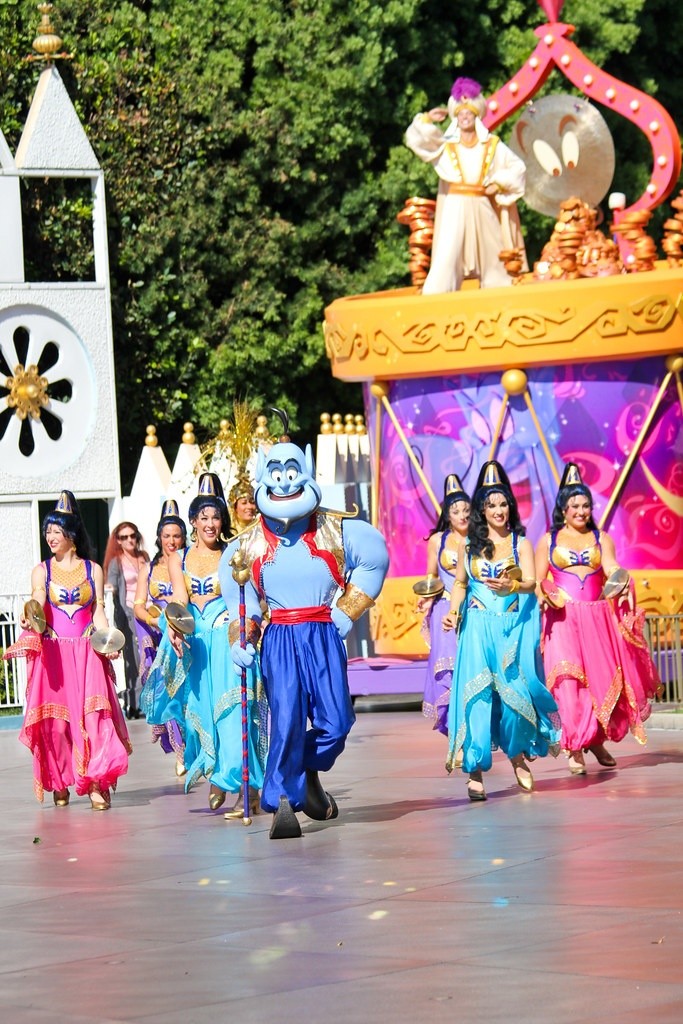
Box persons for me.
[534,463,664,775]
[227,477,256,532]
[139,473,269,818]
[134,499,188,775]
[408,81,526,294]
[417,472,499,752]
[442,459,562,801]
[1,490,133,811]
[104,521,149,719]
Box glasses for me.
[118,533,137,541]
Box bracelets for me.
[146,616,153,627]
[509,580,520,594]
[449,610,462,623]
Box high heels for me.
[89,791,107,811]
[566,749,586,774]
[467,774,486,800]
[224,792,261,820]
[510,756,533,790]
[209,782,225,810]
[53,787,70,805]
[584,744,617,766]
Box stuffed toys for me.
[219,407,389,840]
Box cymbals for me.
[164,602,196,636]
[412,579,445,597]
[540,579,567,611]
[507,94,615,219]
[23,599,47,634]
[90,627,126,657]
[494,565,522,597]
[601,569,632,599]
[147,605,163,618]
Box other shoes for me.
[175,756,186,776]
